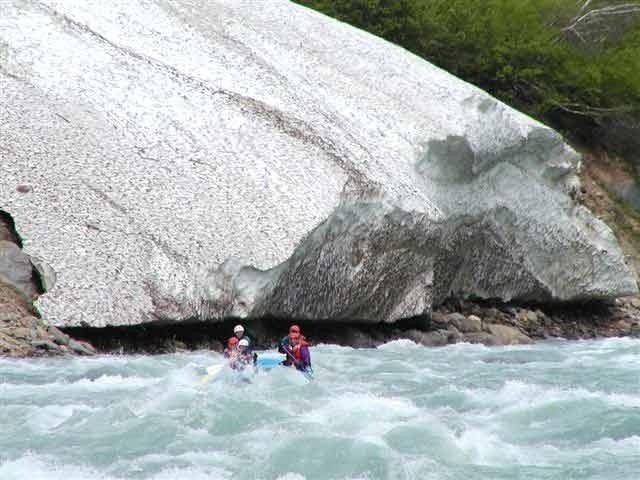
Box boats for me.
[211,357,308,392]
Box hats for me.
[290,326,300,332]
[229,337,238,344]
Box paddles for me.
[281,343,313,379]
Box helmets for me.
[233,325,245,334]
[238,339,249,347]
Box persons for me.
[223,336,240,359]
[278,324,312,371]
[229,338,258,372]
[233,324,253,355]
